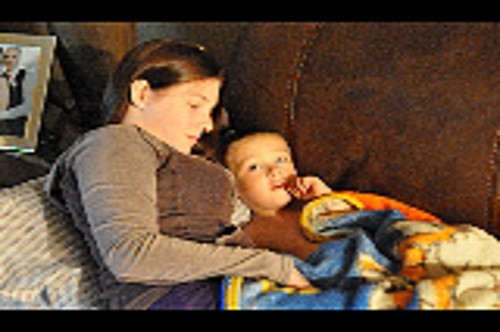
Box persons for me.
[0,44,34,139]
[216,124,500,310]
[44,36,391,311]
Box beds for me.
[0,22,499,252]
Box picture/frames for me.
[0,31,59,152]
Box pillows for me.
[0,166,124,310]
